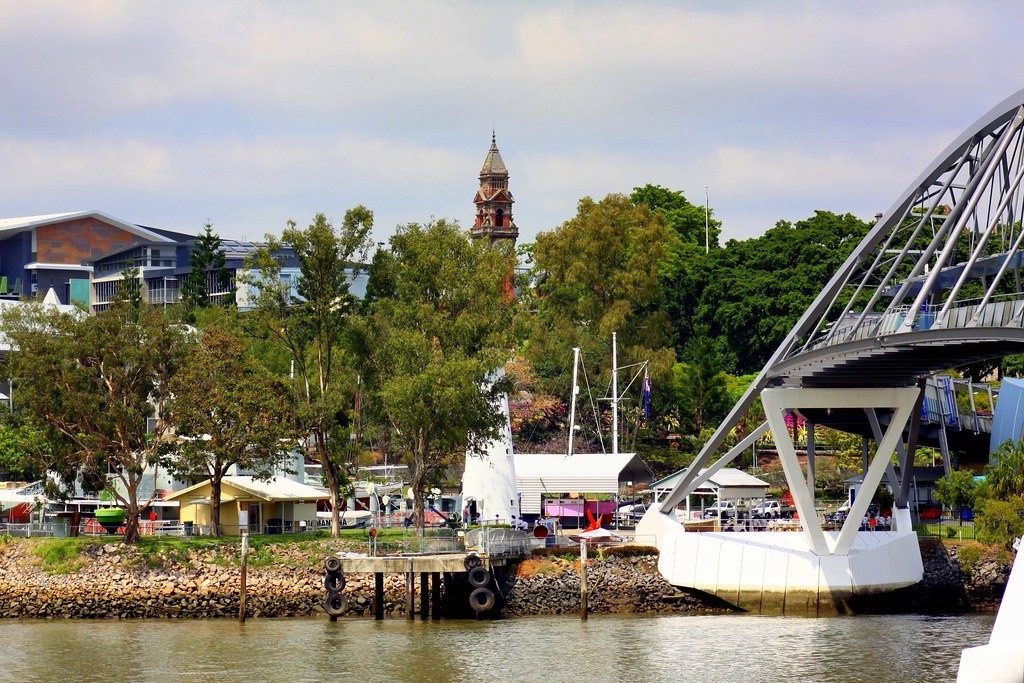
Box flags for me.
[645,368,650,418]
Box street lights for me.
[163,276,180,314]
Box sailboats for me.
[597,332,720,525]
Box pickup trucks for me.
[752,500,797,519]
[705,501,749,518]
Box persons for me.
[863,514,892,531]
[494,514,528,532]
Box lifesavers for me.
[464,555,482,570]
[325,557,341,572]
[469,567,491,588]
[324,572,345,594]
[469,589,494,612]
[324,594,349,617]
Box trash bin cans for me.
[184,521,193,536]
